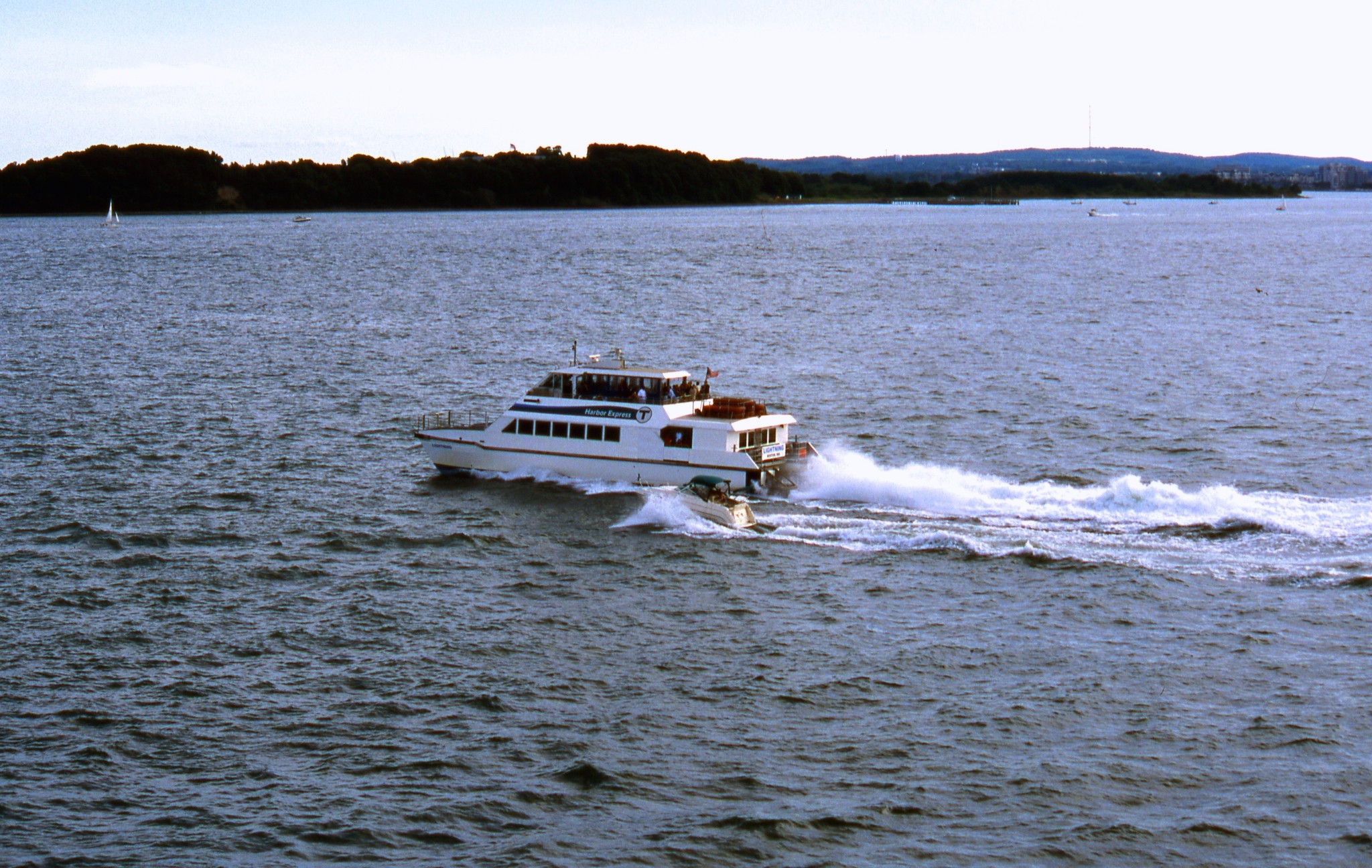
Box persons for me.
[674,428,692,448]
[564,379,710,404]
[706,486,729,508]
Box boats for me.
[413,340,822,499]
[632,474,757,529]
[1087,208,1102,215]
[1208,201,1219,204]
[1071,199,1082,204]
[1123,199,1137,205]
[294,215,311,222]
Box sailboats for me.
[1275,194,1287,210]
[99,198,120,227]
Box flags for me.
[706,367,719,379]
